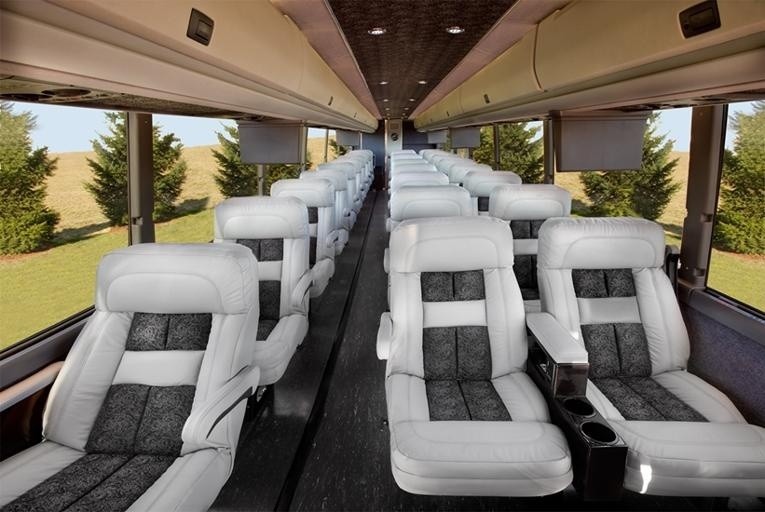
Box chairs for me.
[2,197,309,508]
[376,149,761,512]
[271,150,375,302]
